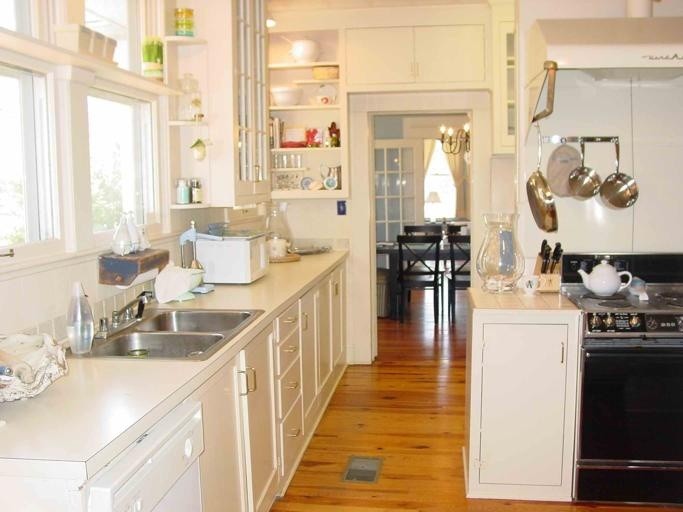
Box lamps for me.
[440,122,470,153]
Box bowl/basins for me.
[174,8,194,36]
[271,87,304,106]
[311,67,339,80]
[317,96,333,105]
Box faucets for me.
[112,297,147,328]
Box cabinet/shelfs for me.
[461,291,583,501]
[277,263,345,498]
[163,1,268,209]
[270,63,350,200]
[195,320,279,512]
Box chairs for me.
[447,236,470,329]
[444,224,470,270]
[405,226,441,267]
[397,236,439,329]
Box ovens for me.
[574,338,681,505]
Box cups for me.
[522,276,547,298]
[273,154,280,168]
[295,155,302,167]
[280,154,287,168]
[461,226,471,235]
[288,154,295,167]
[628,276,646,296]
[266,236,290,257]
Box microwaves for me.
[195,235,269,284]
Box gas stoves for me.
[560,284,683,337]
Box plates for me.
[270,255,301,263]
[287,247,328,255]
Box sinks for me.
[118,310,265,335]
[64,332,231,361]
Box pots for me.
[526,122,558,232]
[568,137,601,198]
[600,141,639,209]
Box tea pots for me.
[474,211,528,296]
[578,259,632,296]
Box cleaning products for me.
[68,281,95,356]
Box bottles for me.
[65,280,94,355]
[267,209,288,260]
[190,178,203,204]
[174,7,195,38]
[175,179,190,204]
[141,37,164,81]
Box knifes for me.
[550,246,564,274]
[542,245,552,274]
[554,242,562,259]
[540,239,548,257]
